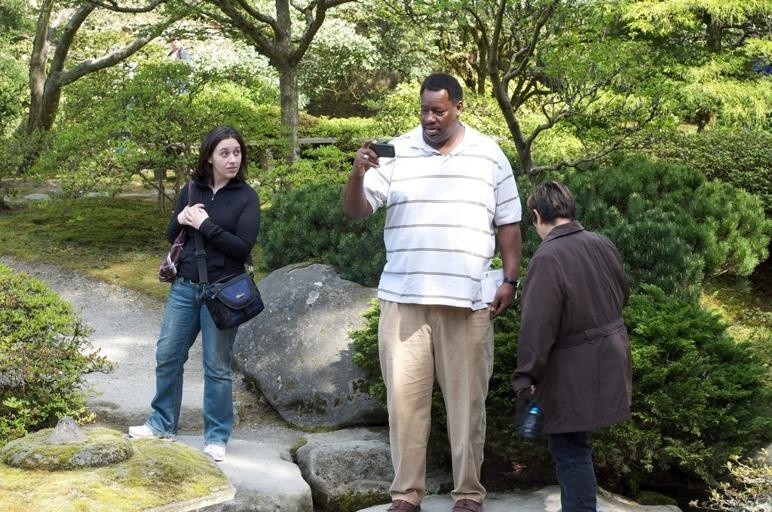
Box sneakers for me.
[203,445,225,461]
[452,499,482,512]
[128,424,173,441]
[388,500,421,512]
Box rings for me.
[363,154,369,159]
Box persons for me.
[342,73,523,512]
[128,125,260,462]
[510,180,632,512]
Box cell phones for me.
[369,144,395,157]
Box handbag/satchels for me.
[203,272,264,330]
[158,227,188,282]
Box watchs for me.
[504,277,521,289]
